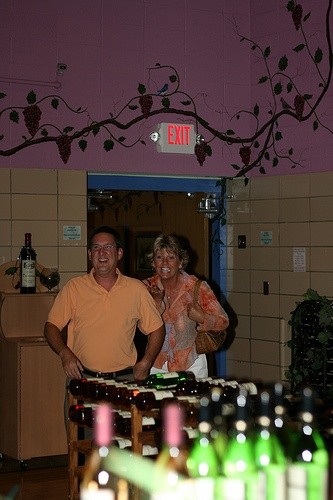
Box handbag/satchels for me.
[193,280,227,354]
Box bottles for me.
[68,370,330,500]
[20,233,36,294]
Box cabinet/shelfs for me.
[67,373,330,500]
[0,260,69,470]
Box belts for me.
[70,364,137,379]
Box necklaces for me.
[166,296,171,300]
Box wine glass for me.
[40,268,60,293]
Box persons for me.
[140,231,229,379]
[44,226,166,468]
